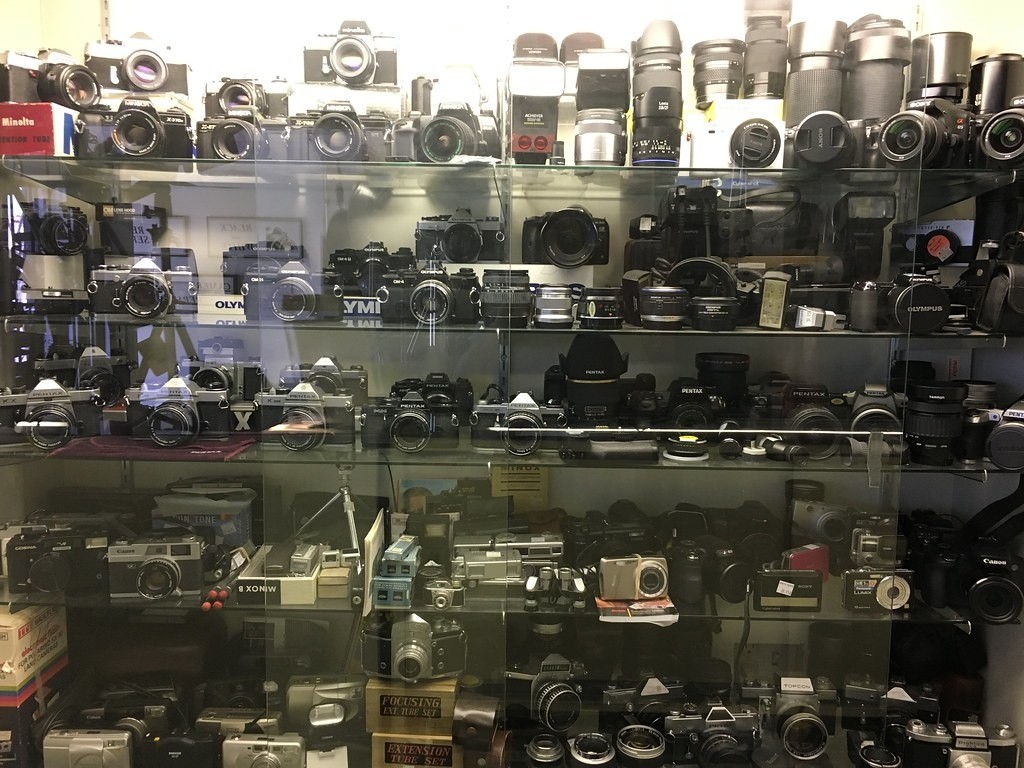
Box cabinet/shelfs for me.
[0,154,1024,768]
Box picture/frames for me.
[206,216,303,263]
[116,213,189,247]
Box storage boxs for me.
[887,219,975,388]
[438,263,593,324]
[372,732,463,768]
[621,99,785,178]
[0,583,68,768]
[18,253,84,290]
[366,676,460,736]
[0,103,81,165]
[237,543,331,605]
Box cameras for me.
[0,0,1024,768]
